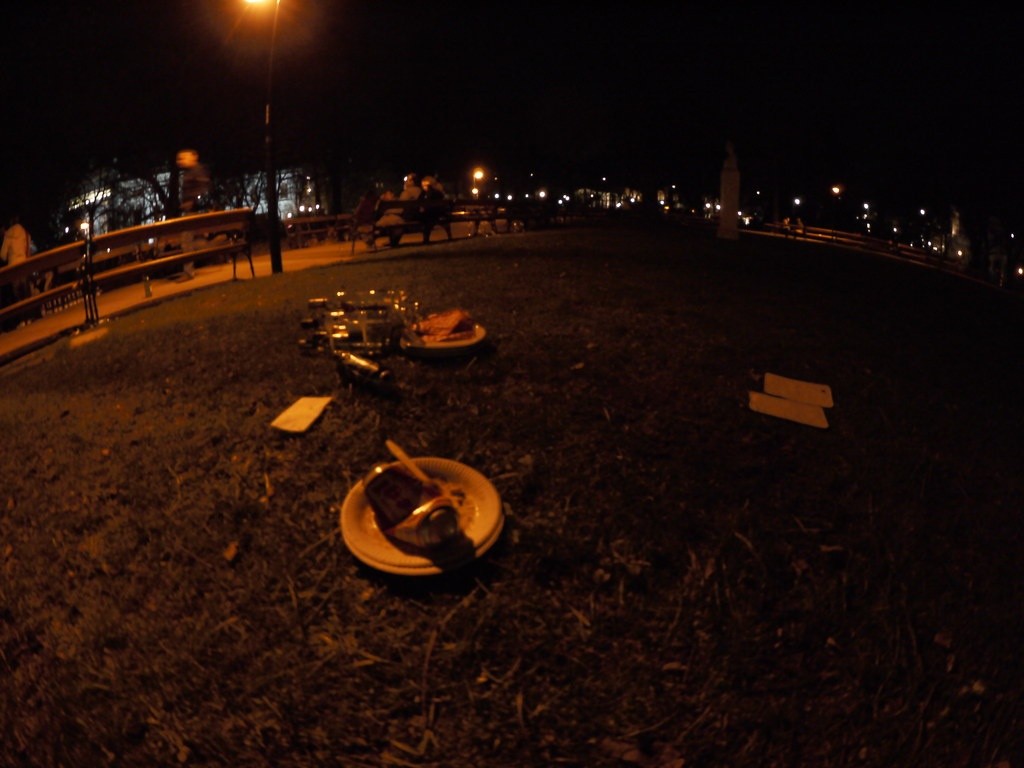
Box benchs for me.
[283,213,356,248]
[88,206,256,323]
[0,238,92,324]
[348,198,464,249]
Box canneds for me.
[361,463,460,551]
[338,351,392,390]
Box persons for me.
[0,213,32,330]
[174,148,211,283]
[353,174,576,245]
[793,218,804,239]
[783,216,790,238]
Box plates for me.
[338,455,504,576]
[403,326,485,352]
